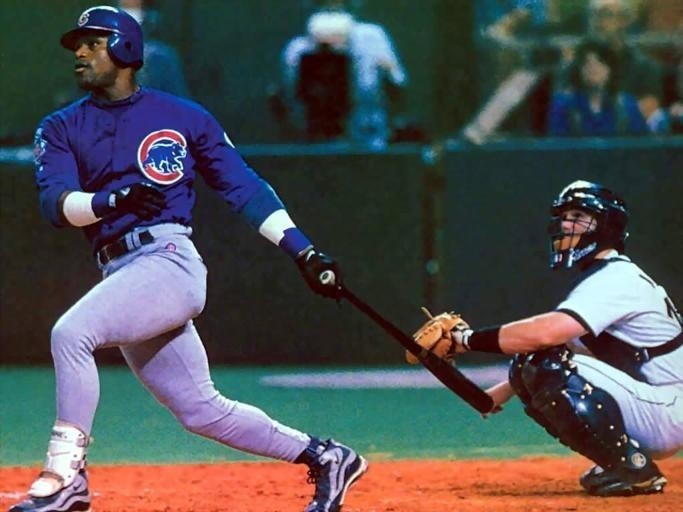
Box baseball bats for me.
[342,285,494,414]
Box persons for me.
[403,179,682,498]
[7,4,368,512]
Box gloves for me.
[108,179,170,222]
[295,249,343,298]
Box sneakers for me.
[41,477,42,480]
[338,459,341,463]
[303,434,368,512]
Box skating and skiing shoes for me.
[7,471,91,511]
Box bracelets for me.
[461,327,503,354]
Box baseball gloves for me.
[405,310,471,362]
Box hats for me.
[574,458,667,498]
[58,3,145,73]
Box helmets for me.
[548,178,629,249]
